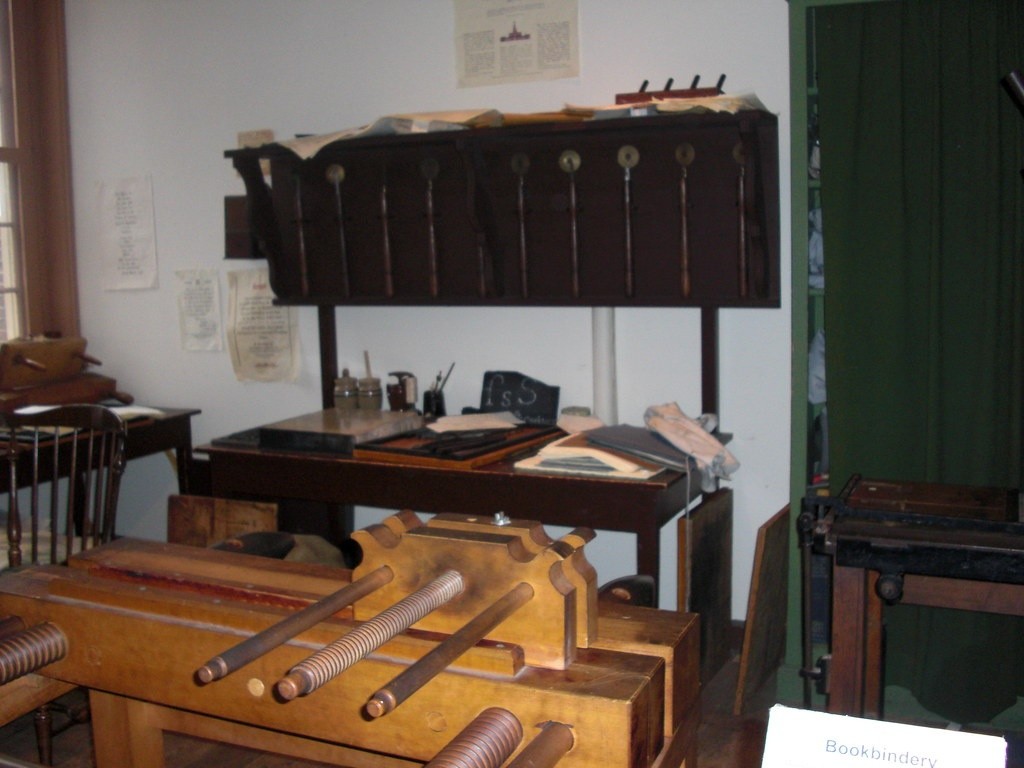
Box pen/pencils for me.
[439,362,454,391]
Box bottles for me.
[333,368,358,407]
[357,378,383,410]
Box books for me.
[513,424,733,482]
[0,405,167,444]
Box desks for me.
[805,470,1022,721]
[189,401,735,614]
[1,405,202,536]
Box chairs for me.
[0,404,127,768]
